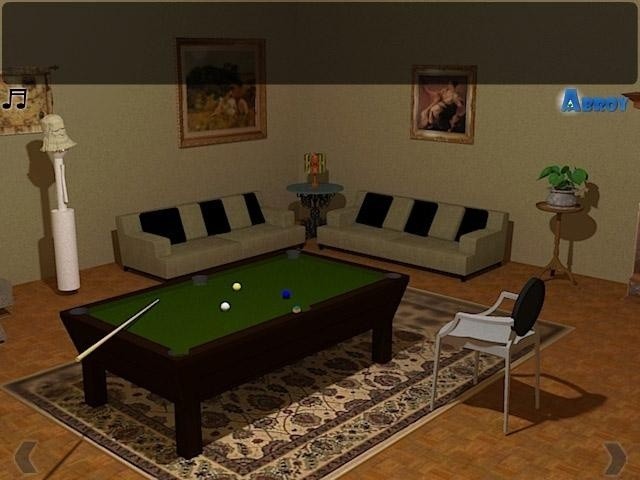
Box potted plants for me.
[536,165,590,208]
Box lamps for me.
[304,152,326,187]
[40,113,81,292]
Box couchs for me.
[316,191,509,282]
[114,190,307,283]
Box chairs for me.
[429,278,545,437]
[0,277,15,342]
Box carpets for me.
[0,286,575,480]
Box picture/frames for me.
[177,37,268,148]
[410,65,478,145]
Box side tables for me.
[287,183,344,240]
[535,202,585,285]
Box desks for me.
[59,246,410,461]
